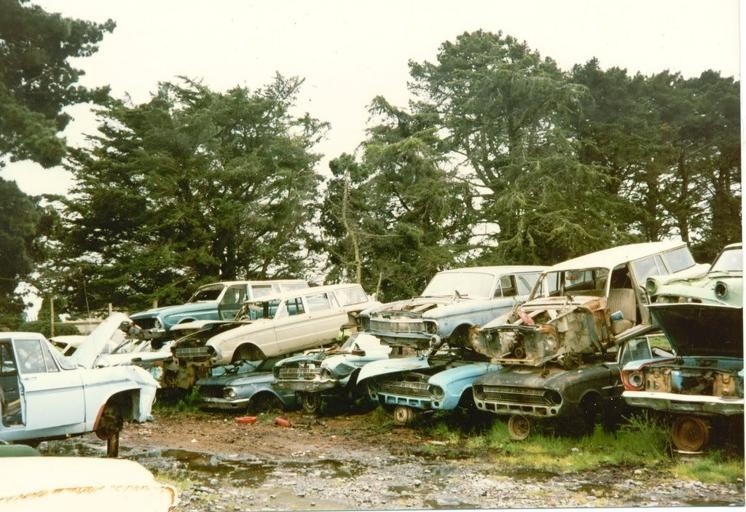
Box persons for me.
[133,338,171,423]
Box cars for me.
[44,234,743,435]
[0,307,165,444]
